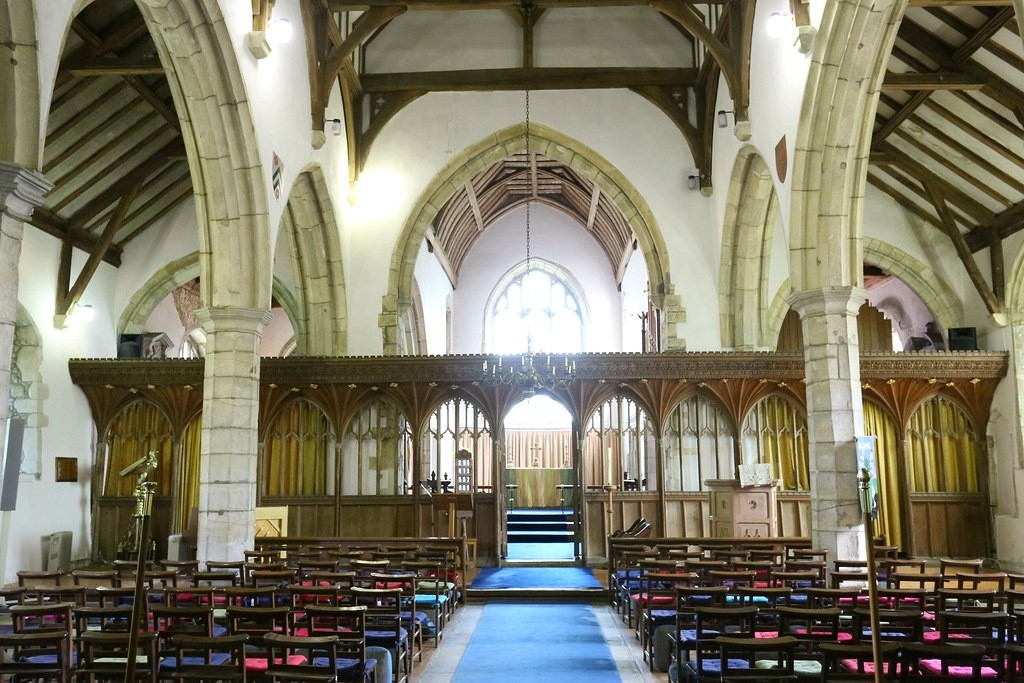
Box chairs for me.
[609,542,1024,683]
[0,540,459,683]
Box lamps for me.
[322,118,341,134]
[147,333,175,359]
[688,174,705,188]
[717,110,737,127]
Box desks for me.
[556,484,580,511]
[255,537,467,603]
[472,483,517,513]
[609,538,814,586]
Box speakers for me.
[947,325,977,350]
[117,333,143,359]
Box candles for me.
[482,354,576,375]
